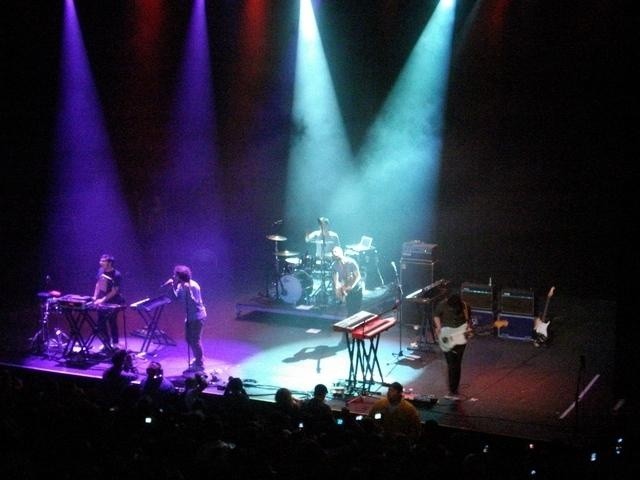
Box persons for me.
[140,361,163,394]
[90,255,123,350]
[332,246,363,317]
[102,349,140,400]
[168,265,207,366]
[275,388,300,421]
[220,377,249,406]
[300,384,335,433]
[305,217,340,256]
[433,288,472,401]
[367,382,420,440]
[177,373,208,428]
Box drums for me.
[277,268,313,304]
[284,257,302,269]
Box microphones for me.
[316,217,324,225]
[160,278,173,288]
[98,273,113,282]
[272,218,285,226]
[391,261,400,275]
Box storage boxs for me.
[501,288,536,316]
[498,314,535,341]
[463,283,494,310]
[465,311,495,336]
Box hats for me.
[147,361,160,374]
[315,384,328,394]
[112,349,126,362]
[447,293,460,308]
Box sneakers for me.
[453,394,460,401]
[444,392,452,399]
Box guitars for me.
[533,287,556,346]
[266,235,288,241]
[438,318,510,352]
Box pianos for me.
[130,294,173,312]
[333,311,379,332]
[353,316,397,339]
[403,278,454,304]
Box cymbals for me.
[272,250,300,256]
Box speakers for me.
[565,354,607,387]
[562,398,595,428]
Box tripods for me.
[386,277,427,366]
